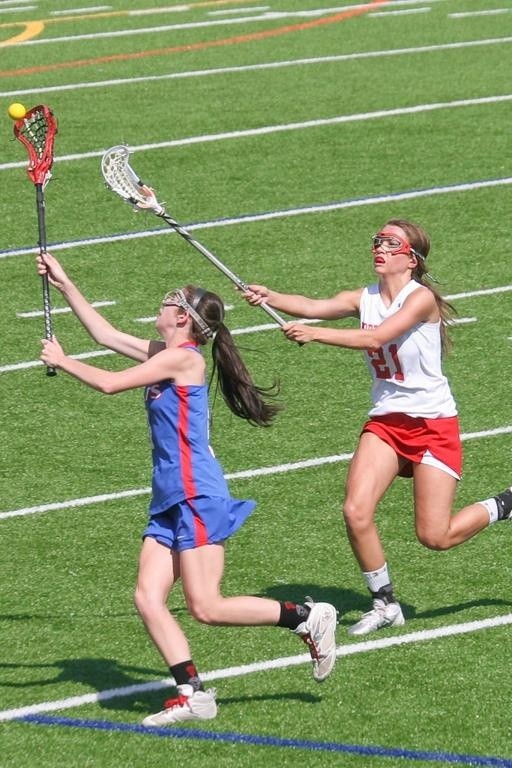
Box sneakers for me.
[347,598,405,636]
[289,595,340,682]
[141,683,219,729]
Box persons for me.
[231,219,511,636]
[35,250,339,731]
